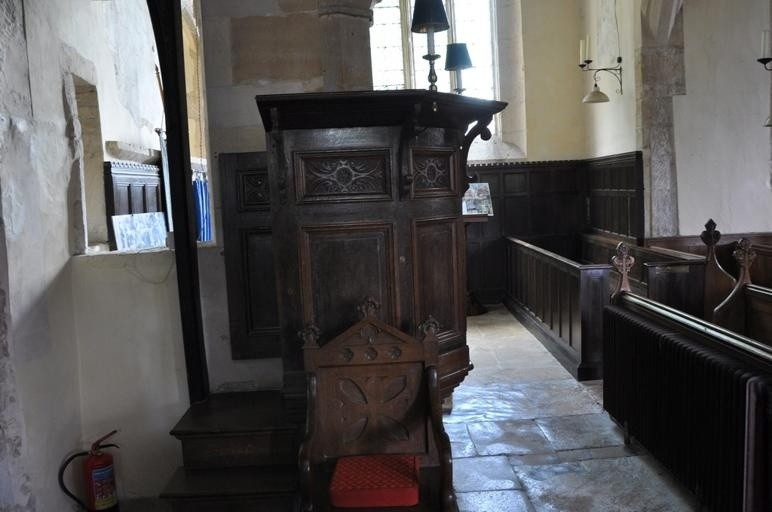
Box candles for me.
[580,39,585,64]
[761,28,768,58]
[586,34,591,61]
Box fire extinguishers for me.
[58,430,120,512]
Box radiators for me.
[601,303,772,511]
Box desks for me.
[158,391,305,512]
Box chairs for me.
[298,298,458,511]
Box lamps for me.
[411,0,449,91]
[446,44,471,95]
[582,67,623,103]
